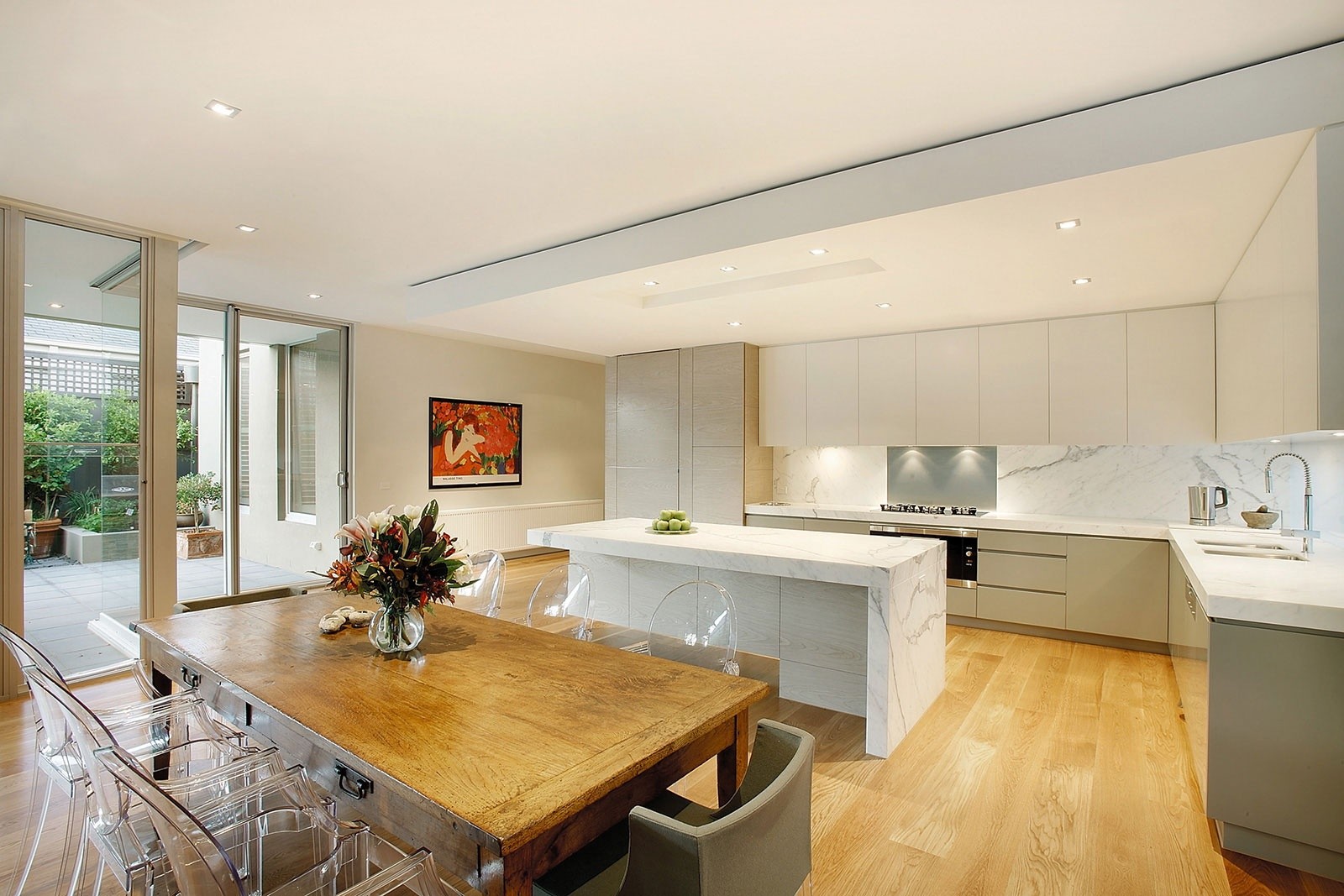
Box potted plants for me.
[23,389,94,560]
[176,471,223,561]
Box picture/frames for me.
[428,396,523,491]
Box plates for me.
[646,526,698,534]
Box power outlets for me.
[918,574,927,593]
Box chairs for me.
[529,718,816,896]
[619,579,742,675]
[0,625,465,896]
[514,562,594,639]
[434,548,508,622]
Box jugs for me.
[1188,485,1227,526]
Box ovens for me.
[869,523,978,589]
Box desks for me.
[129,586,769,896]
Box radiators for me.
[434,498,604,558]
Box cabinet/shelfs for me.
[975,530,1344,884]
[754,116,1344,448]
[604,341,775,526]
[746,514,870,536]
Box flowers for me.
[303,498,482,650]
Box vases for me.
[367,598,425,654]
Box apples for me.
[651,509,690,531]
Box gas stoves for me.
[870,502,990,518]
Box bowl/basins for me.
[1241,512,1279,528]
